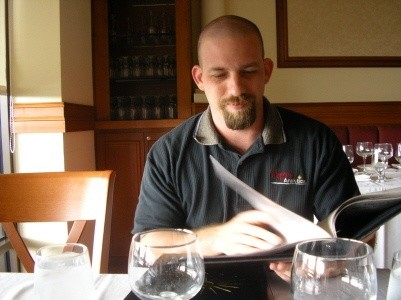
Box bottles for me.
[108,54,175,79]
[111,95,177,119]
[109,11,176,46]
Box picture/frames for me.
[275,0,401,69]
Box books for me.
[205,154,401,264]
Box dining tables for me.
[354,165,401,269]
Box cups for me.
[395,143,401,164]
[342,145,354,164]
[128,228,205,300]
[290,237,378,300]
[386,250,401,300]
[35,243,93,300]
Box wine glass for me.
[357,141,394,182]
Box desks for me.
[0,267,131,300]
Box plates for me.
[391,164,401,169]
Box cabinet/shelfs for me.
[92,0,193,132]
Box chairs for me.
[0,169,117,273]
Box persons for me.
[125,14,377,300]
